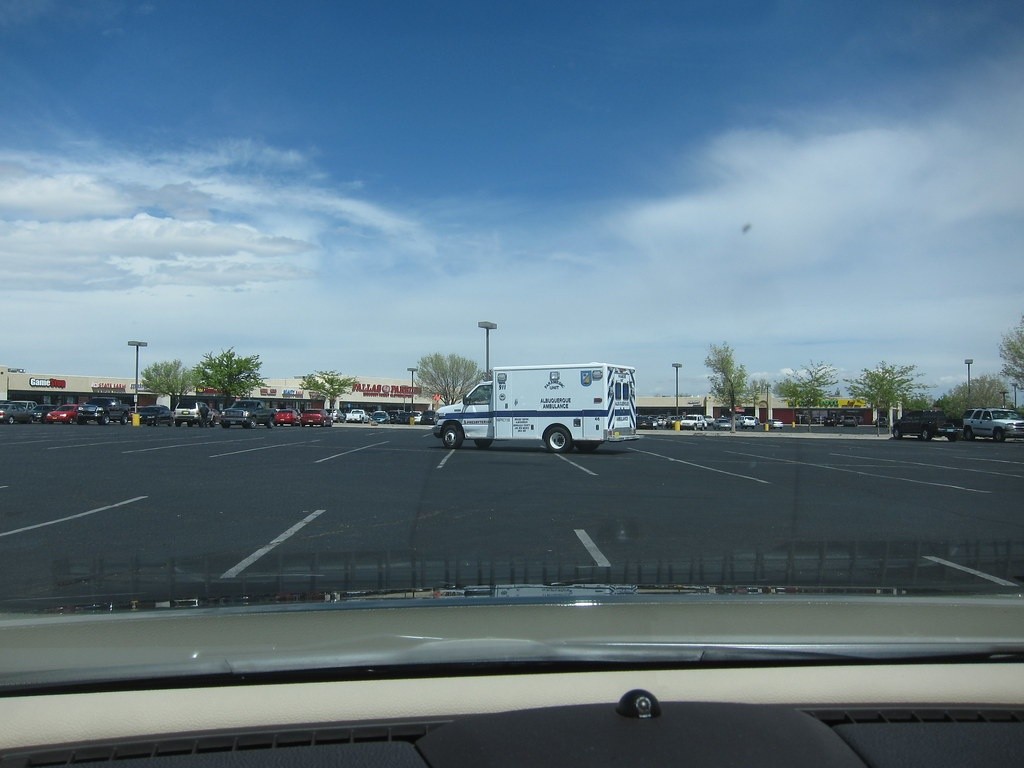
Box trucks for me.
[432,361,644,452]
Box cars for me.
[636,414,668,430]
[372,411,390,423]
[0,404,35,424]
[713,418,732,430]
[128,406,145,423]
[275,409,301,426]
[300,409,334,427]
[137,405,174,426]
[290,409,301,426]
[46,404,79,424]
[30,405,55,424]
[388,410,435,425]
[767,419,784,429]
[326,408,345,423]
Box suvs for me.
[736,416,756,429]
[843,416,858,426]
[963,407,1024,441]
[667,416,680,430]
[681,415,705,430]
[824,417,837,427]
[11,401,38,411]
[174,400,217,428]
[875,417,888,427]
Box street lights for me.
[766,384,771,423]
[477,321,497,381]
[1000,390,1009,408]
[1012,383,1018,409]
[672,364,683,421]
[128,341,147,413]
[965,359,973,409]
[407,368,418,425]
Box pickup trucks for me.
[76,397,131,425]
[218,400,276,428]
[704,415,715,424]
[892,410,964,442]
[345,409,370,424]
